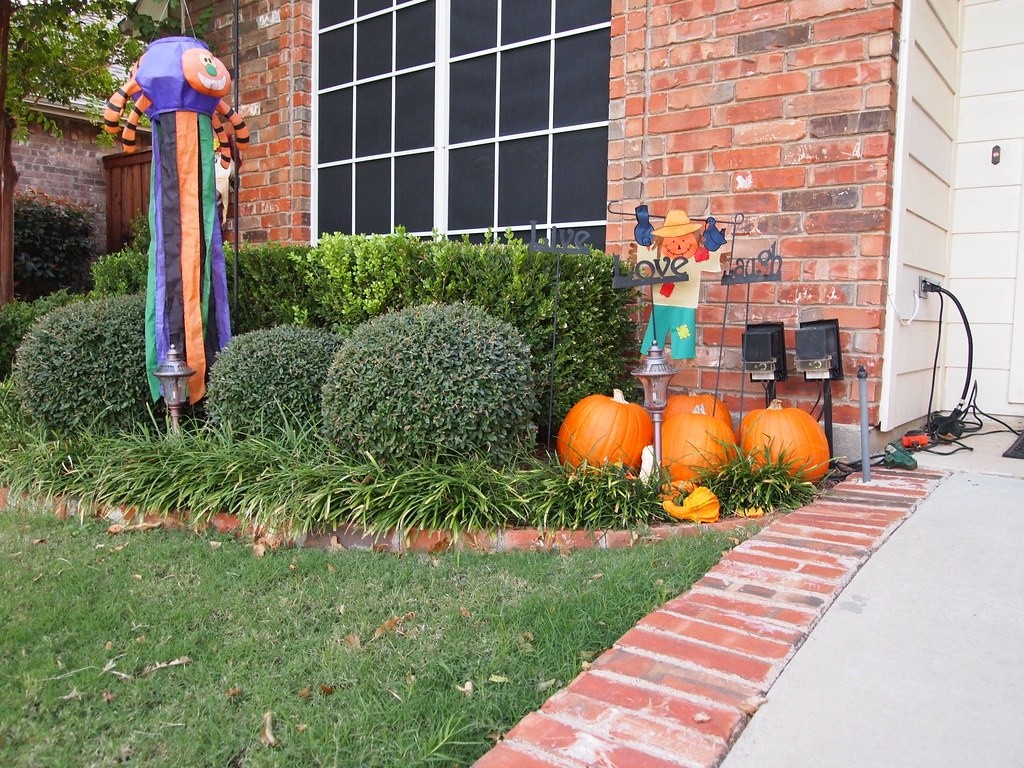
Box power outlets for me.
[918,276,928,299]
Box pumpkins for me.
[556,389,830,524]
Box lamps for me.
[631,340,678,411]
[151,344,196,406]
